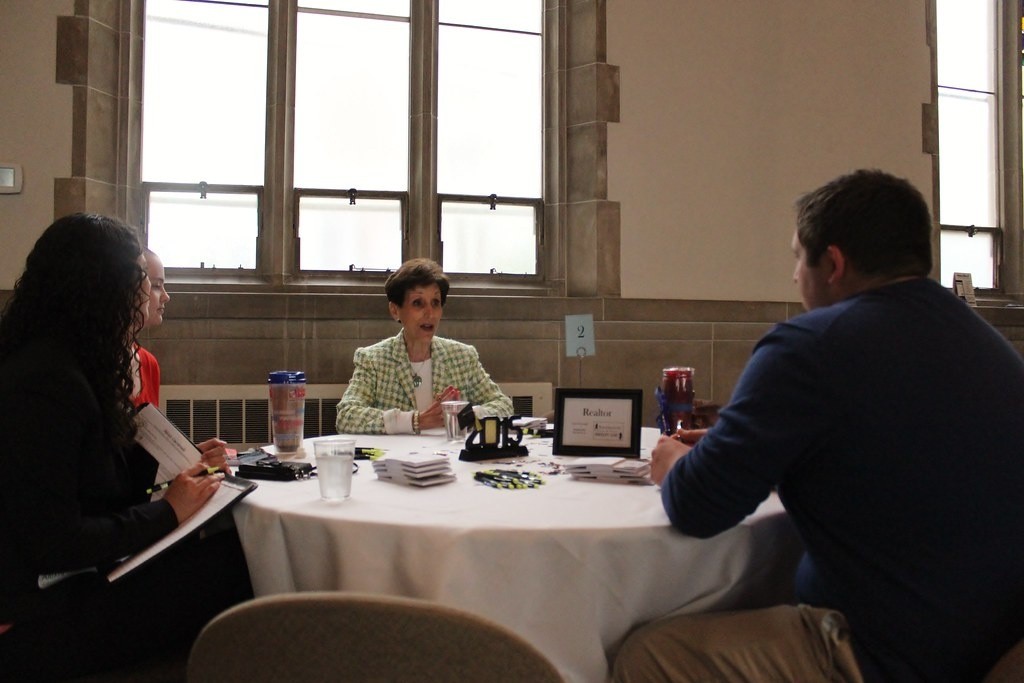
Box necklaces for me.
[408,345,431,389]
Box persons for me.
[122,240,236,474]
[604,168,1020,683]
[1,210,251,680]
[331,257,512,438]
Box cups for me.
[441,400,470,443]
[267,370,307,452]
[314,439,355,500]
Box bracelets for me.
[414,410,422,436]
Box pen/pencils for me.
[355,447,387,460]
[473,467,546,491]
[523,426,553,437]
[654,384,671,436]
[147,465,221,495]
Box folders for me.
[97,400,259,583]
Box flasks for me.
[660,367,696,442]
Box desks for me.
[222,423,812,683]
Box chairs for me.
[177,588,561,683]
